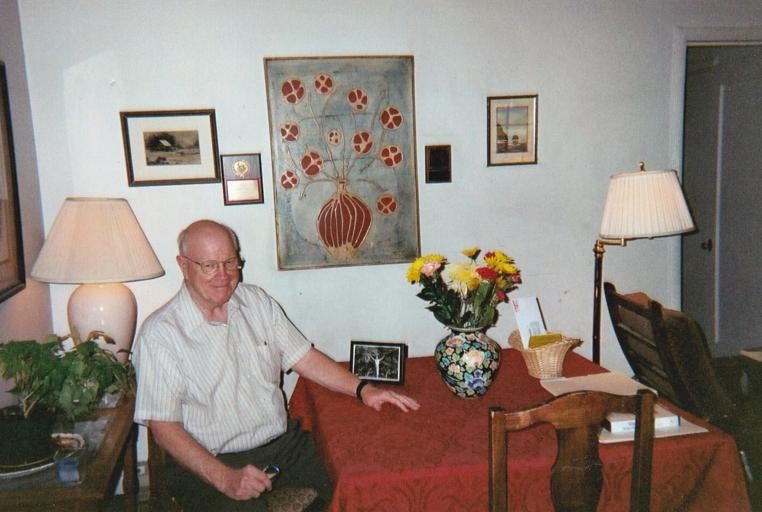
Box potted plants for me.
[0,332,135,490]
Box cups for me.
[53,447,87,486]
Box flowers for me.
[408,247,522,327]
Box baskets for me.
[508,330,584,381]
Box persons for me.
[135,219,419,507]
[370,355,385,377]
[509,134,520,147]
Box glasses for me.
[179,256,244,276]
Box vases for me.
[434,328,501,398]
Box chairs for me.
[604,281,762,487]
[488,389,655,511]
[147,426,319,512]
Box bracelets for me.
[356,381,367,401]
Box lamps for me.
[31,196,165,365]
[590,161,697,363]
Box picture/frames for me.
[263,55,421,272]
[487,93,537,166]
[118,110,221,187]
[0,61,26,306]
[349,340,408,385]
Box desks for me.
[741,347,761,397]
[1,378,139,512]
[286,350,752,511]
